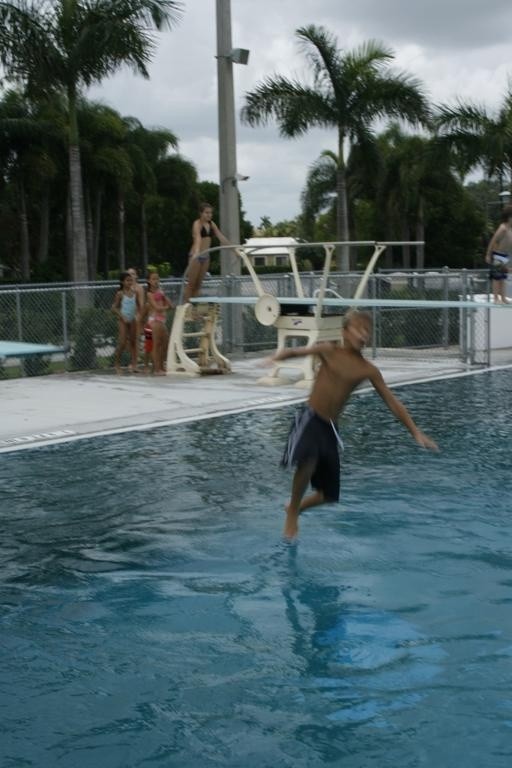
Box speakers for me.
[229,47,249,64]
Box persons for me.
[126,267,147,370]
[484,205,512,305]
[145,271,175,377]
[181,201,240,321]
[249,307,443,539]
[137,301,166,372]
[111,272,141,374]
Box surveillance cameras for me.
[235,173,250,183]
